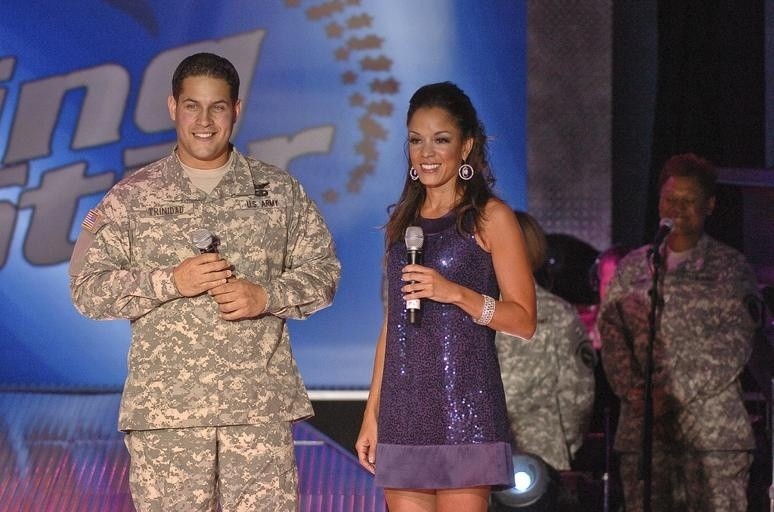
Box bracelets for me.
[471,292,496,327]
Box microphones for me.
[404,226,424,324]
[646,217,673,257]
[191,229,228,284]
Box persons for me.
[489,209,596,511]
[68,52,341,511]
[355,82,537,511]
[593,153,765,512]
